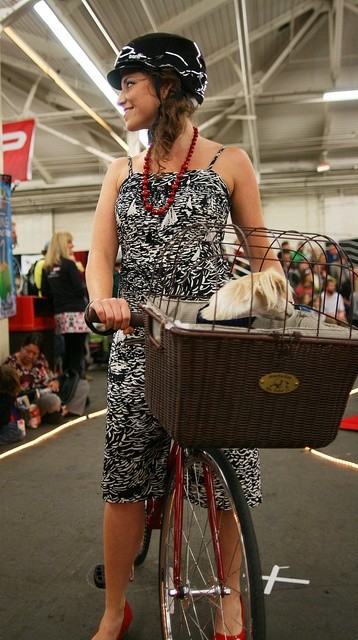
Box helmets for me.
[107,32,207,104]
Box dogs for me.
[195,266,327,329]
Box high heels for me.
[212,592,246,639]
[116,603,132,640]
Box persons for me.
[83,32,298,640]
[230,241,248,275]
[100,252,123,369]
[39,231,89,412]
[275,238,358,331]
[5,333,64,425]
[29,242,66,374]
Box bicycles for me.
[90,224,358,640]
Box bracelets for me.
[82,296,115,336]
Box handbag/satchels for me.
[55,367,79,405]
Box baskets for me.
[140,222,357,450]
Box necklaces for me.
[142,127,198,215]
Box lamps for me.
[32,0,152,149]
[322,90,358,102]
[317,165,330,173]
[81,0,120,57]
